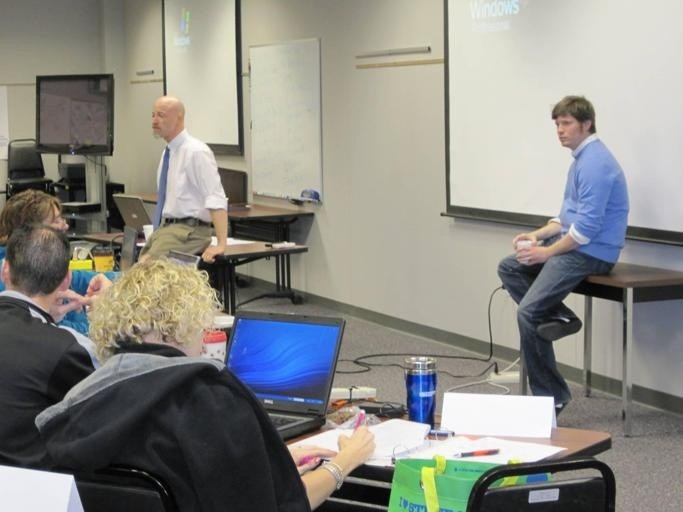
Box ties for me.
[153,145,171,232]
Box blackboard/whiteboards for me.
[248,37,323,203]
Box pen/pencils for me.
[353,409,365,433]
[460,449,498,457]
[429,429,454,436]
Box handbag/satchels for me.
[386,456,551,512]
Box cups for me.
[143,225,153,243]
[213,314,235,345]
[513,239,531,265]
[93,245,116,274]
[200,331,227,363]
[402,355,437,432]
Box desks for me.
[519,262,683,436]
[81,233,308,319]
[131,193,314,306]
[279,399,611,484]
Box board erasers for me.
[301,189,318,200]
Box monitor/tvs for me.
[35,72,114,156]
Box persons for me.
[0,189,116,338]
[498,95,629,416]
[138,96,229,265]
[34,256,377,512]
[0,221,113,473]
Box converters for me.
[359,401,404,417]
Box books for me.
[322,435,570,469]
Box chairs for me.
[466,457,616,512]
[5,138,53,202]
[70,466,178,512]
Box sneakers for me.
[555,399,571,418]
[537,316,583,342]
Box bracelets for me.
[319,462,344,490]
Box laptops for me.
[118,225,138,275]
[225,310,346,442]
[112,193,152,237]
